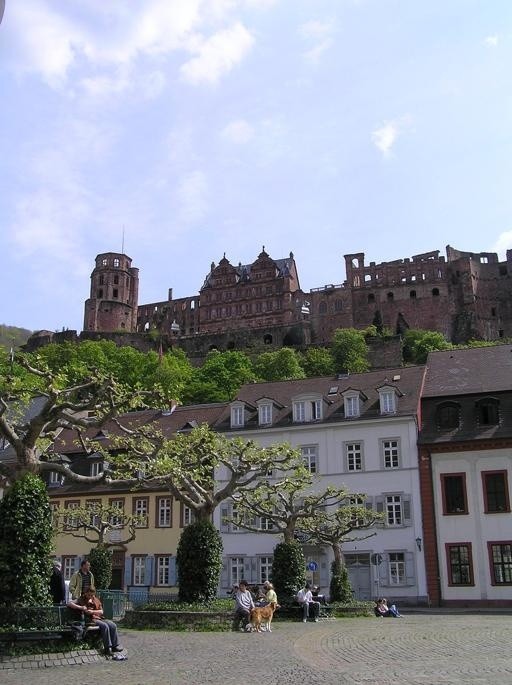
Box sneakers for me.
[104,645,124,656]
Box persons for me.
[377,599,404,618]
[232,581,256,631]
[69,560,94,600]
[297,583,320,622]
[67,585,123,655]
[48,561,65,605]
[311,586,320,596]
[254,580,277,613]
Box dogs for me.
[245,600,281,634]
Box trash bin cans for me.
[103,592,114,619]
[318,594,325,605]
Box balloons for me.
[309,562,317,570]
